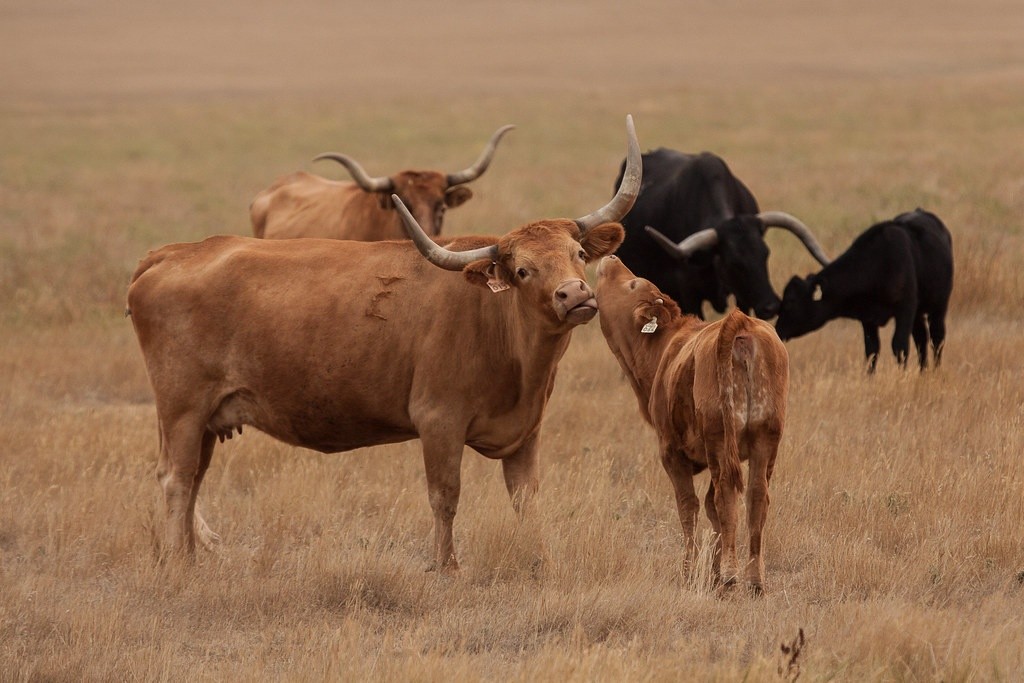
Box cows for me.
[775,207,953,373]
[596,256,789,598]
[125,112,643,573]
[613,146,831,321]
